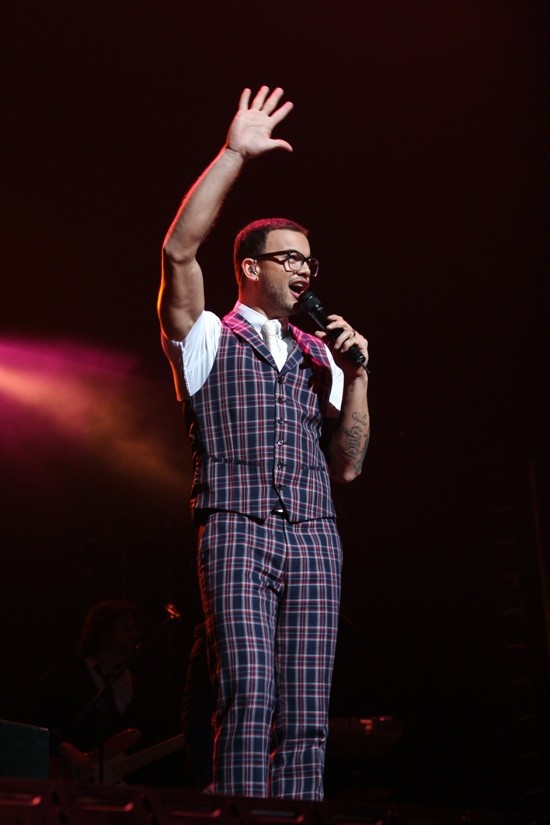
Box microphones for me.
[300,292,371,375]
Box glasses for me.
[239,249,320,281]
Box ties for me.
[261,320,287,373]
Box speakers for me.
[0,719,50,775]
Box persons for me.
[157,87,371,802]
[33,600,191,785]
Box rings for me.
[352,329,356,336]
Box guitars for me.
[47,727,187,785]
